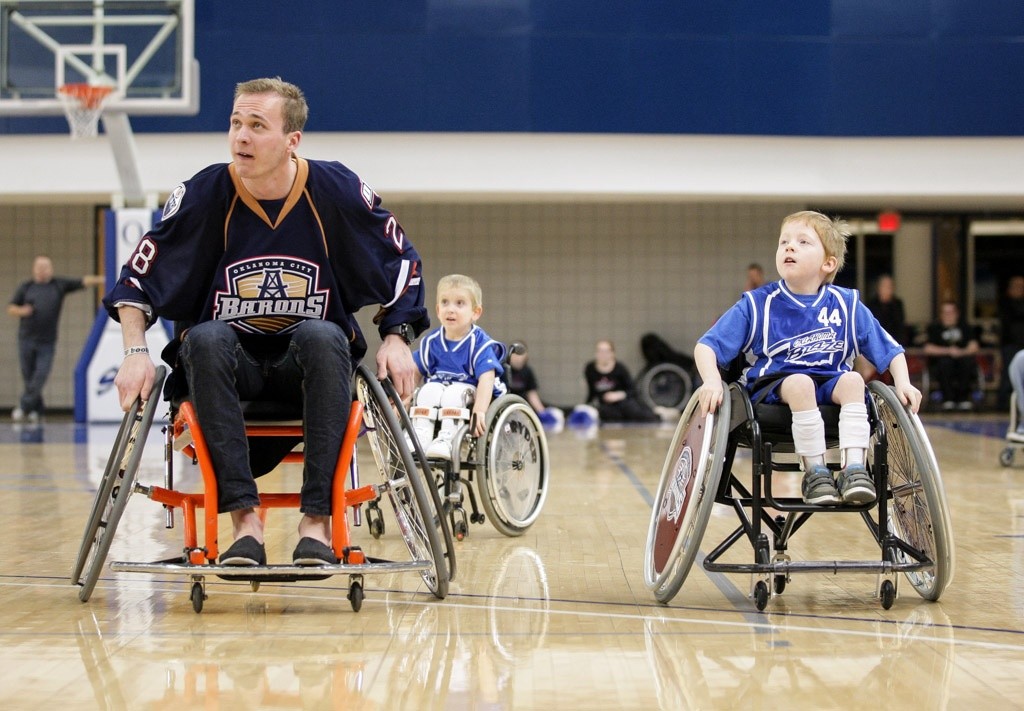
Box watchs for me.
[389,322,415,346]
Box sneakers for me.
[293,537,336,565]
[801,466,842,504]
[218,535,267,565]
[405,435,425,454]
[837,465,877,503]
[424,432,450,462]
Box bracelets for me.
[124,346,149,360]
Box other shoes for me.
[27,409,39,425]
[12,404,25,422]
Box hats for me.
[509,340,528,354]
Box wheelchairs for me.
[998,392,1024,467]
[643,351,956,612]
[910,347,987,413]
[633,333,697,418]
[366,341,551,541]
[69,352,458,612]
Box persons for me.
[923,301,979,410]
[500,342,545,413]
[1005,350,1024,443]
[402,274,507,461]
[102,78,430,567]
[7,256,106,420]
[864,276,904,347]
[744,263,763,291]
[584,339,680,423]
[694,210,922,505]
